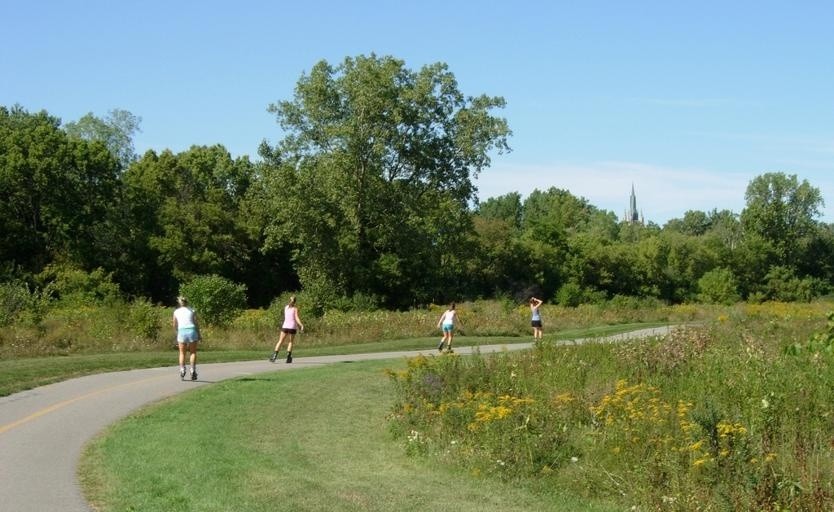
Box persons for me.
[171,295,203,381]
[529,296,543,343]
[268,296,305,363]
[436,302,463,353]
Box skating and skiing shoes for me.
[285,352,293,363]
[269,351,278,362]
[179,367,186,381]
[189,367,198,380]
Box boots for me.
[437,341,444,352]
[446,345,454,354]
